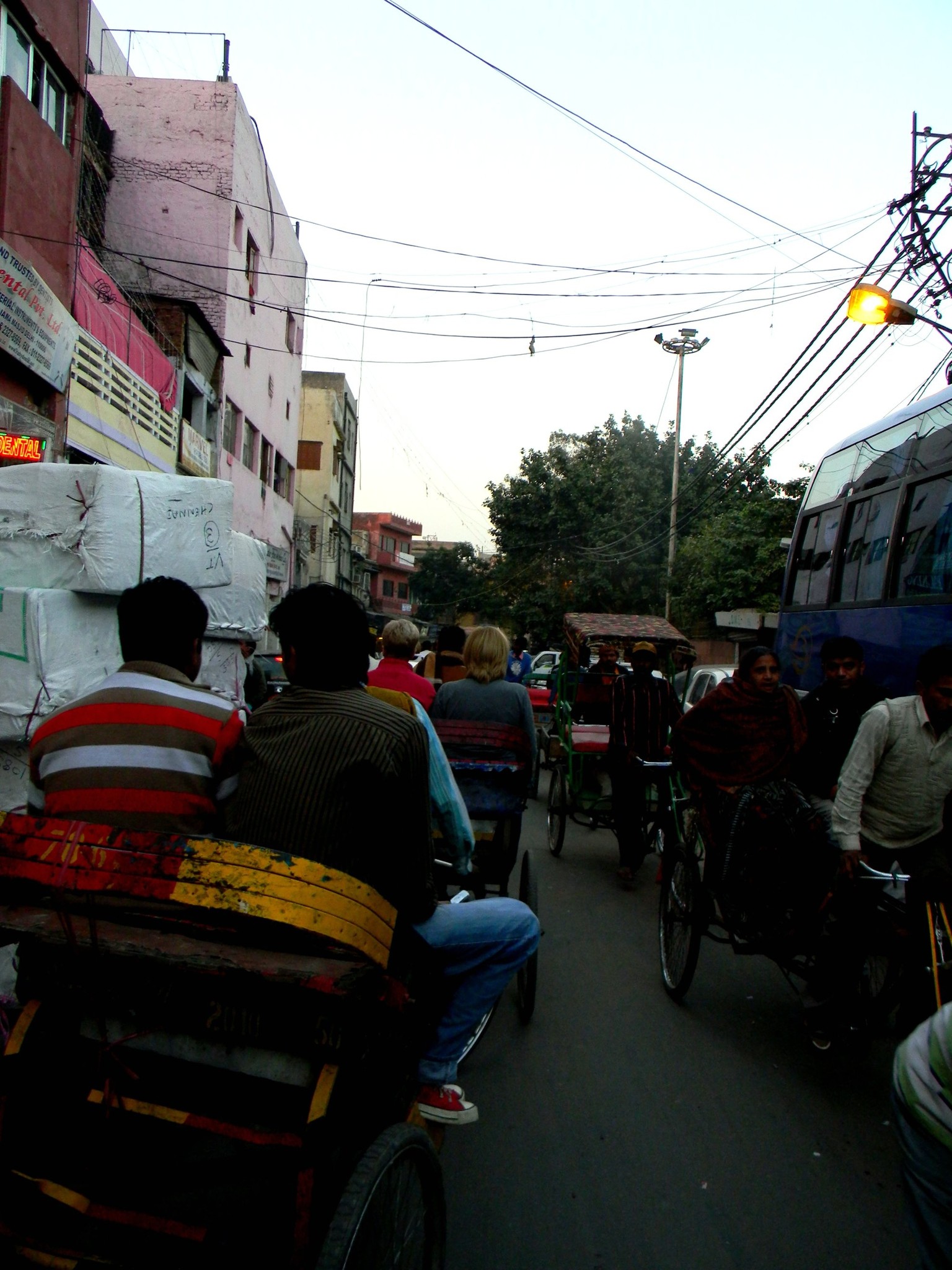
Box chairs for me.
[562,673,617,752]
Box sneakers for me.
[416,1081,479,1125]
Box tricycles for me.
[0,852,500,1270]
[540,611,952,1043]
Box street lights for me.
[654,326,711,626]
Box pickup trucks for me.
[529,650,634,689]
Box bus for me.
[771,382,952,706]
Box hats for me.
[632,640,658,659]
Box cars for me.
[589,654,739,715]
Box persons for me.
[889,999,952,1270]
[548,638,696,727]
[804,641,952,1048]
[670,634,897,848]
[240,584,542,1126]
[242,601,538,876]
[611,639,683,882]
[28,574,247,839]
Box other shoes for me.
[615,860,637,881]
[802,1006,833,1050]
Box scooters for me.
[533,652,562,735]
[364,610,397,661]
